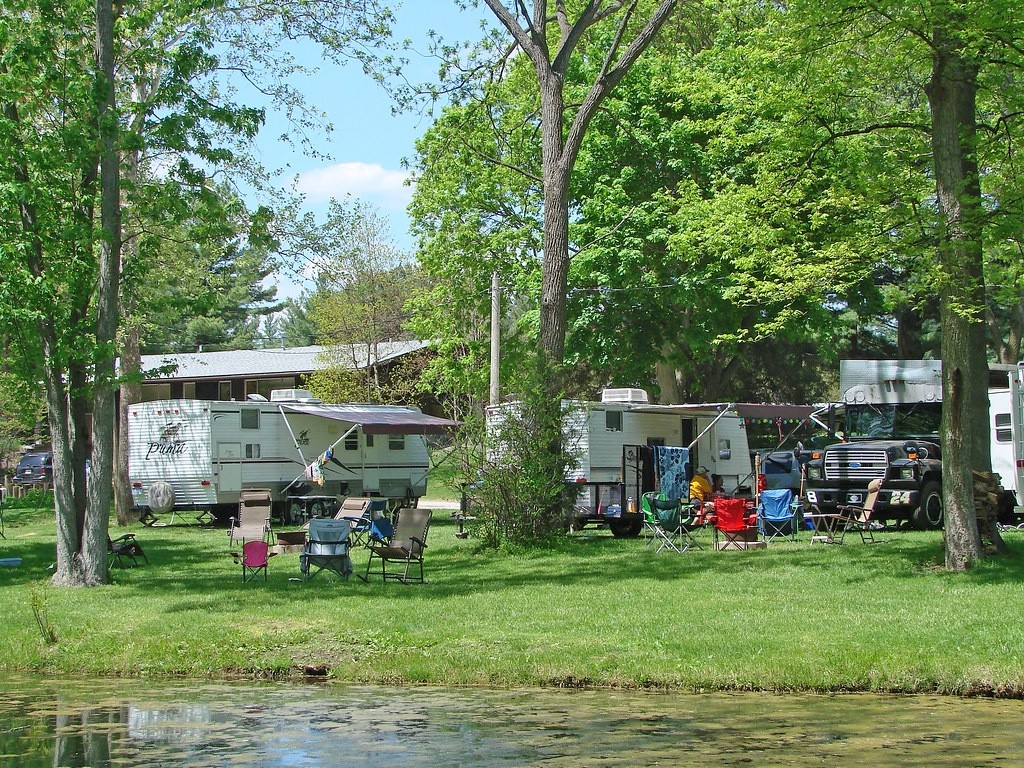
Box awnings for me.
[278,405,463,494]
[632,402,815,495]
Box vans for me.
[13,450,53,489]
[749,446,819,498]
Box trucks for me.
[801,359,1023,529]
[484,387,813,538]
[127,389,466,526]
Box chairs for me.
[226,487,274,549]
[334,496,390,550]
[356,508,432,585]
[639,489,875,556]
[240,540,268,584]
[301,518,353,584]
[107,532,149,570]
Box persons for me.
[689,466,713,525]
[711,473,726,493]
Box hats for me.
[696,465,710,474]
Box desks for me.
[804,513,840,545]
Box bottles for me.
[626,496,635,513]
